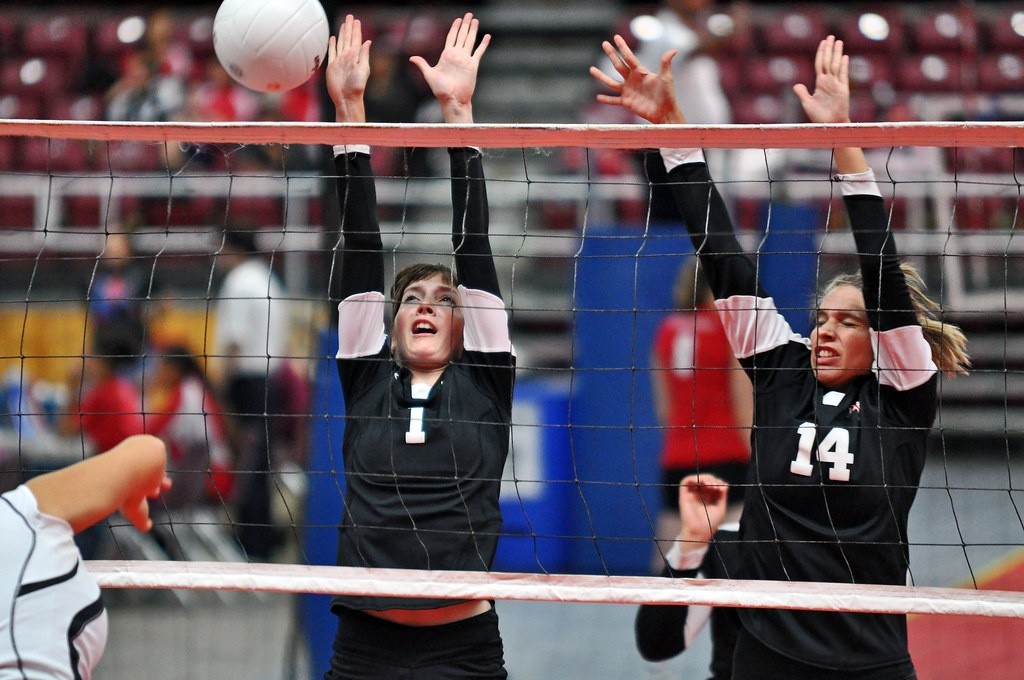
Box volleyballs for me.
[211,0,330,94]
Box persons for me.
[592,30,967,680]
[321,14,518,679]
[207,225,290,565]
[0,1,464,680]
